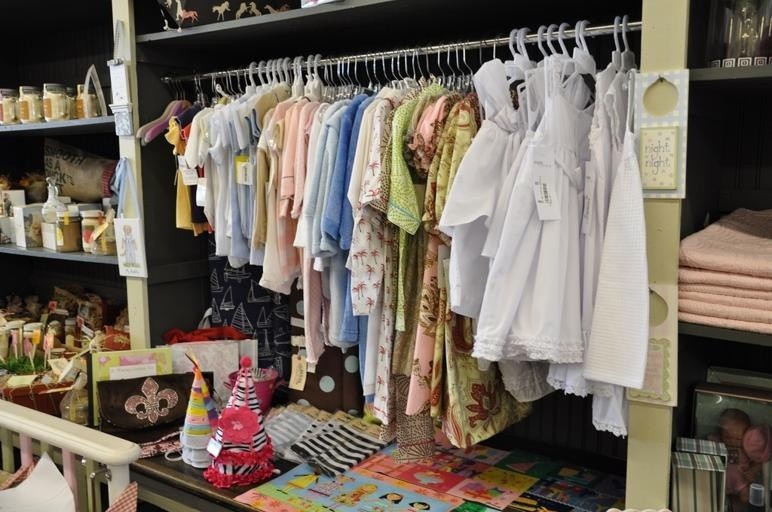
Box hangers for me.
[137,15,637,148]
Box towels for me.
[678,209,772,333]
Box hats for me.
[164,347,220,469]
[202,356,274,489]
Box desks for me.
[91,394,279,511]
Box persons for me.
[705,407,763,512]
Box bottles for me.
[41,175,70,223]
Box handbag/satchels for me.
[95,371,214,444]
[154,326,258,412]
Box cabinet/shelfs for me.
[0,0,151,351]
[623,0,772,512]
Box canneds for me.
[55,210,104,252]
[7,316,80,350]
[0,82,98,128]
